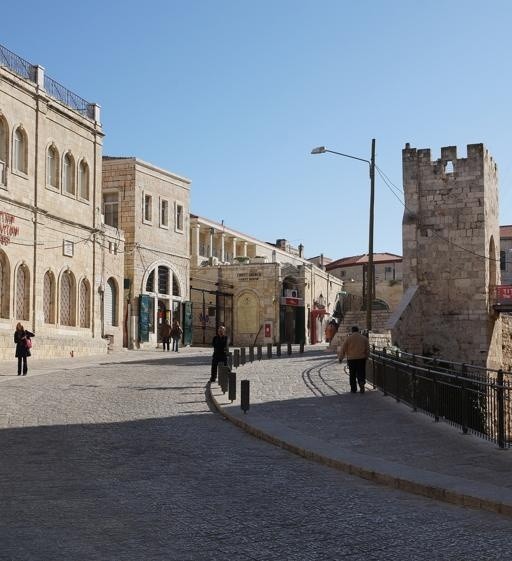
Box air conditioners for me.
[284,289,297,298]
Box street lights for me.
[311,139,375,330]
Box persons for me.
[210,325,229,382]
[159,319,171,352]
[14,322,36,376]
[170,320,183,352]
[338,325,370,393]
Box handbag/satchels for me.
[24,337,32,348]
